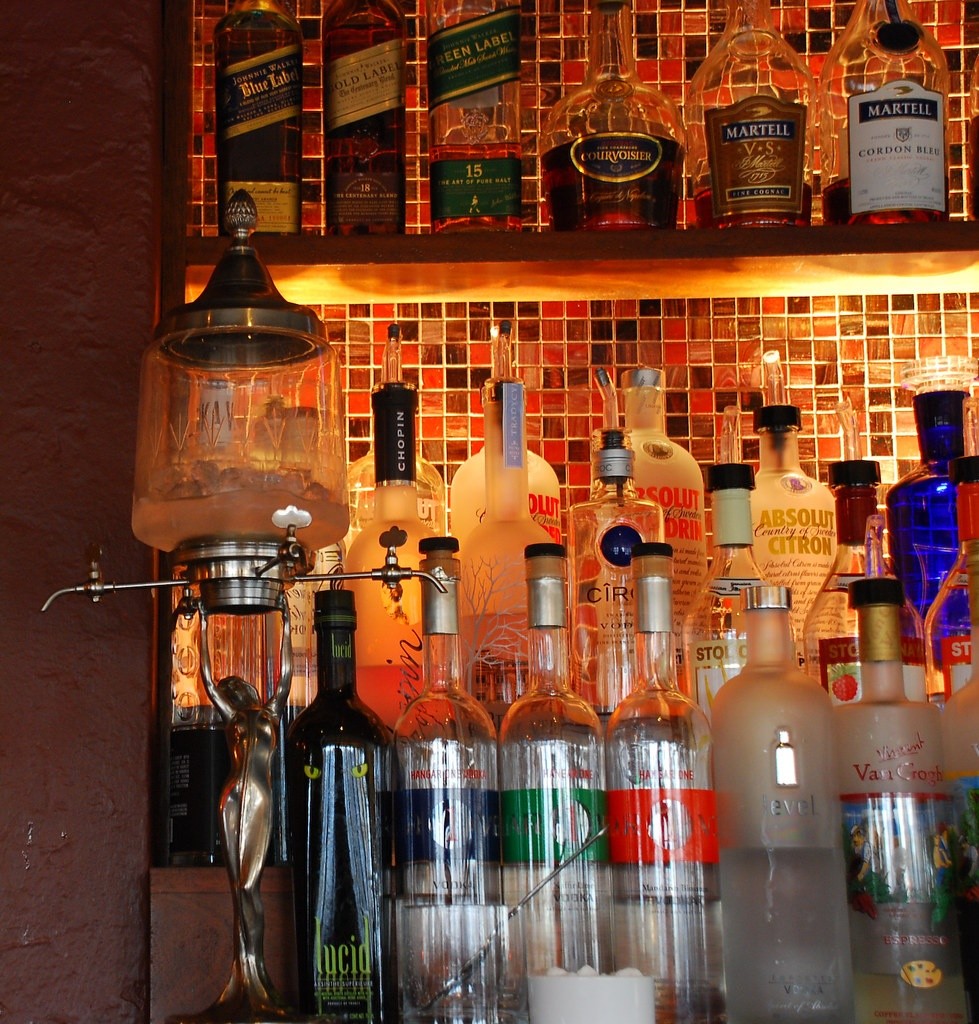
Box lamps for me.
[41,187,449,1023]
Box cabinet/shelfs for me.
[145,0,979,1024]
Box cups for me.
[402,905,528,1024]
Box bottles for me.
[713,586,856,1024]
[285,590,393,1024]
[450,320,563,735]
[395,538,501,1024]
[425,0,522,234]
[568,369,709,733]
[607,542,721,1024]
[802,356,978,714]
[819,0,951,225]
[214,0,304,236]
[683,0,818,230]
[540,0,685,234]
[942,539,979,1024]
[502,544,615,1024]
[835,578,961,1024]
[684,348,837,720]
[322,0,406,236]
[340,325,452,735]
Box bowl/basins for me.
[527,975,655,1024]
